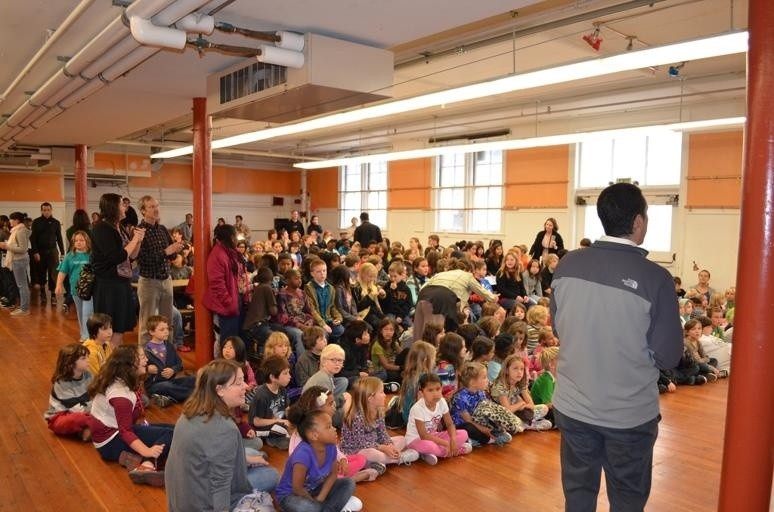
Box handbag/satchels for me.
[75,264,96,300]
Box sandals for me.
[119,450,164,486]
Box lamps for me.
[149,0,749,159]
[582,23,686,77]
[292,76,746,170]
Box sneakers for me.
[384,382,400,393]
[535,420,552,430]
[266,438,289,450]
[399,449,437,465]
[496,432,512,446]
[361,462,386,481]
[10,307,30,316]
[459,443,472,454]
[151,394,171,407]
[695,373,717,385]
[340,496,362,512]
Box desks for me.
[130,278,190,290]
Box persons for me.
[1,193,567,511]
[658,270,736,393]
[550,184,685,511]
